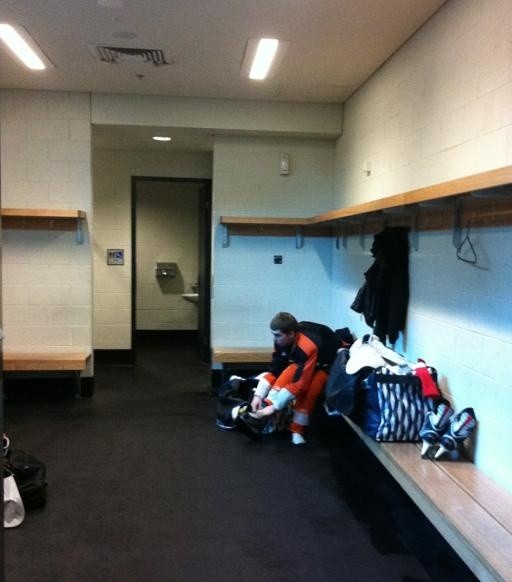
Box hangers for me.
[456,219,477,264]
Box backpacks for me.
[4,452,48,513]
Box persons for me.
[247,312,341,445]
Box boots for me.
[418,403,456,458]
[432,407,477,461]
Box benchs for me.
[1,352,91,401]
[341,411,511,582]
[212,348,275,380]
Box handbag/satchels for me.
[216,378,294,446]
[363,366,440,444]
